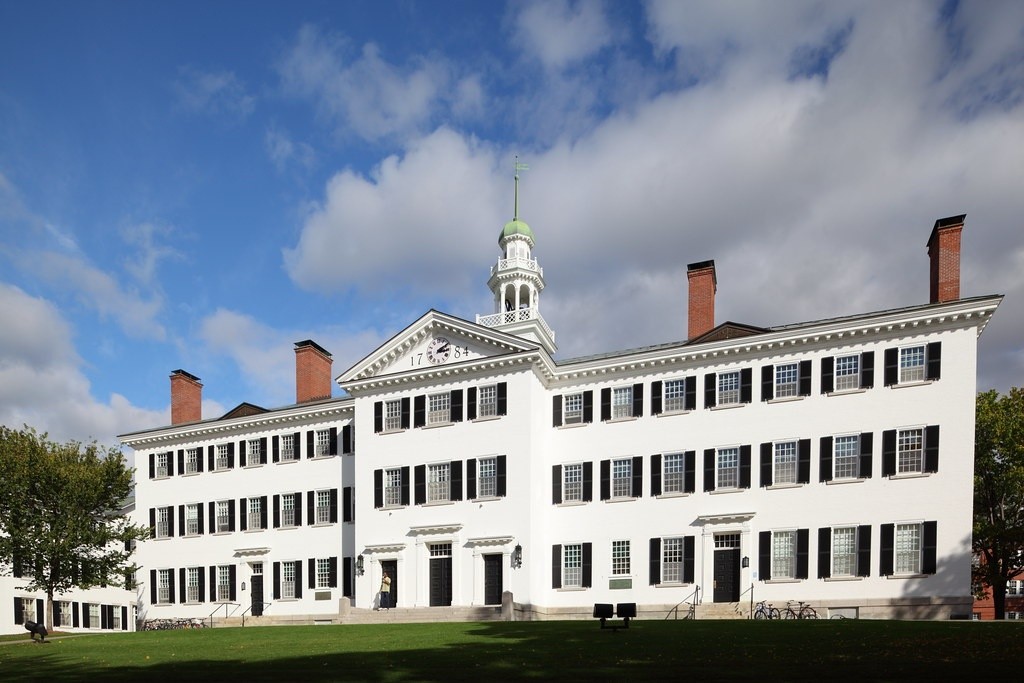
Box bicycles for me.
[779,600,817,620]
[140,617,210,631]
[754,600,781,619]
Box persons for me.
[378,571,391,613]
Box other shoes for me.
[382,608,388,611]
[378,607,383,610]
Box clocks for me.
[427,337,450,366]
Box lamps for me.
[515,542,523,569]
[742,555,750,569]
[25,621,49,642]
[594,603,637,630]
[357,554,365,576]
[242,582,245,590]
